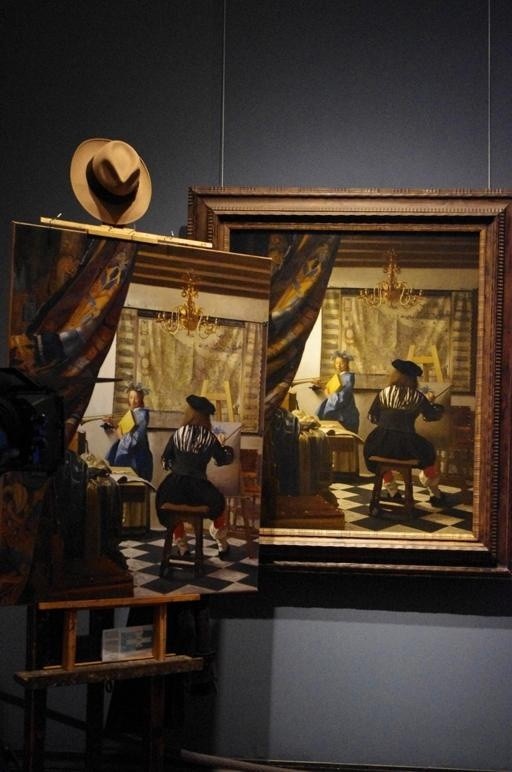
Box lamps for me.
[155,266,221,341]
[357,244,426,313]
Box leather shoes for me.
[169,549,192,560]
[218,542,231,560]
[381,490,402,502]
[428,491,454,508]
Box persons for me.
[156,394,237,563]
[315,351,360,435]
[364,358,453,508]
[102,381,153,481]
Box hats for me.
[66,134,155,230]
[391,358,423,378]
[186,394,218,416]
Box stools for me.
[156,497,212,584]
[366,454,421,522]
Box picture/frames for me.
[184,184,512,583]
